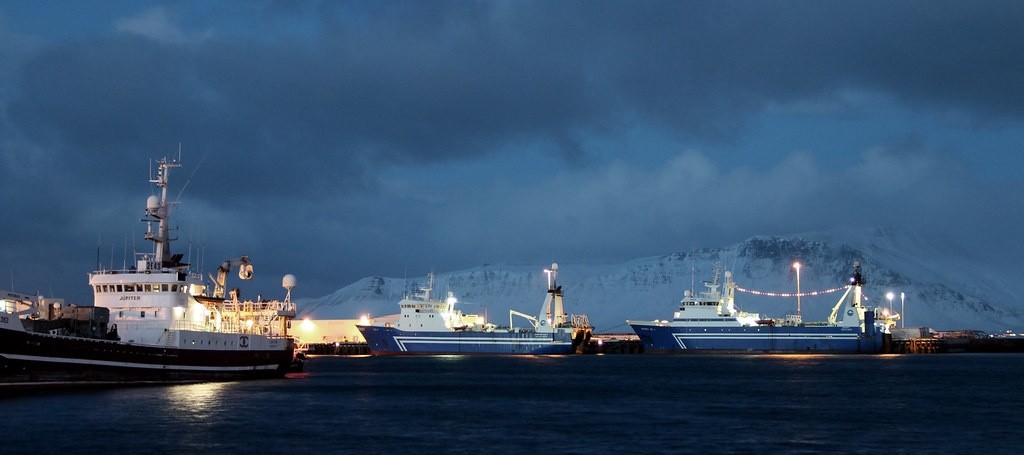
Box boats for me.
[0,142,302,378]
[624,256,896,354]
[357,262,595,355]
